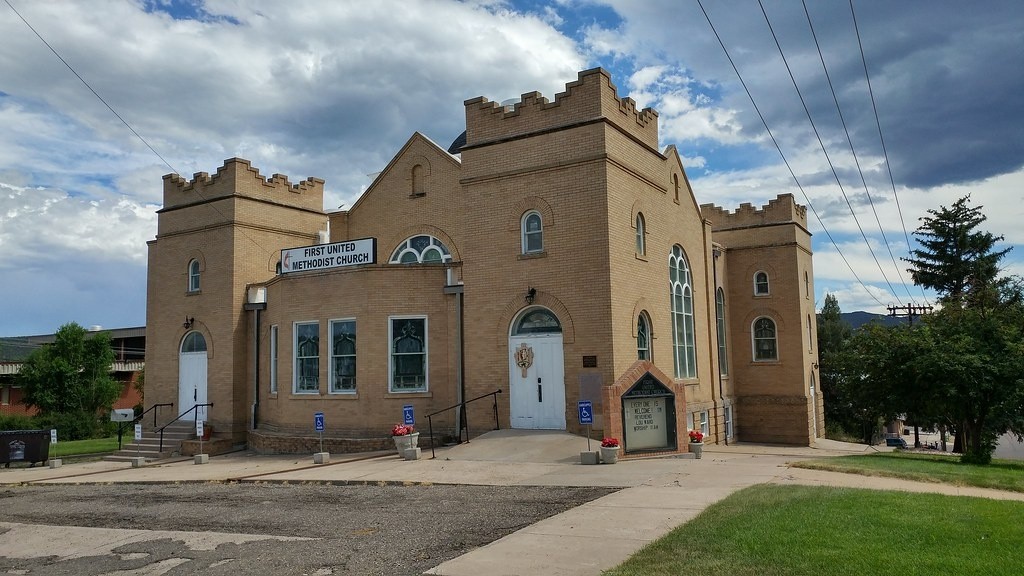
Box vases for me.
[393,432,419,457]
[600,446,620,464]
[688,442,704,459]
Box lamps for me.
[183,316,194,329]
[525,288,536,305]
[812,360,818,369]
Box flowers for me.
[688,431,703,443]
[391,424,414,436]
[601,438,619,447]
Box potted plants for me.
[196,423,212,441]
